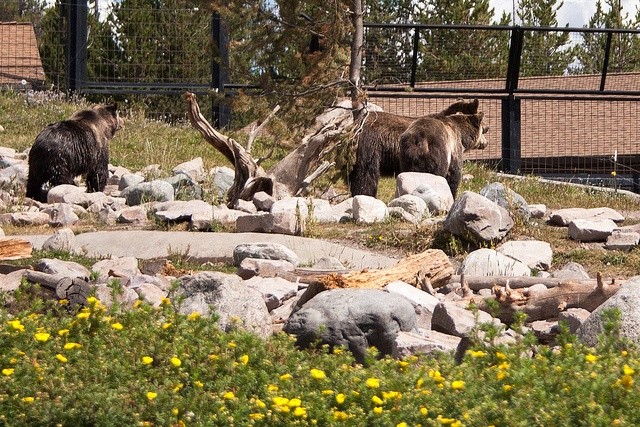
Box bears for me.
[25,102,126,204]
[343,97,490,197]
[397,111,490,205]
